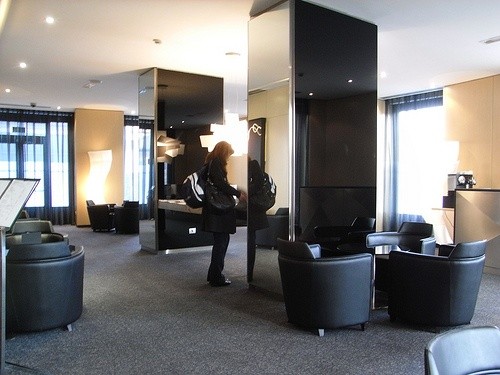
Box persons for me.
[197,141,247,287]
[248,156,268,282]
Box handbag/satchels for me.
[252,172,275,212]
[203,183,235,214]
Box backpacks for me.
[183,165,206,209]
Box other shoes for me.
[224,278,232,284]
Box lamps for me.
[87,150,113,196]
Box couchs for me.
[86,199,140,234]
[6,211,85,341]
[276,222,500,375]
